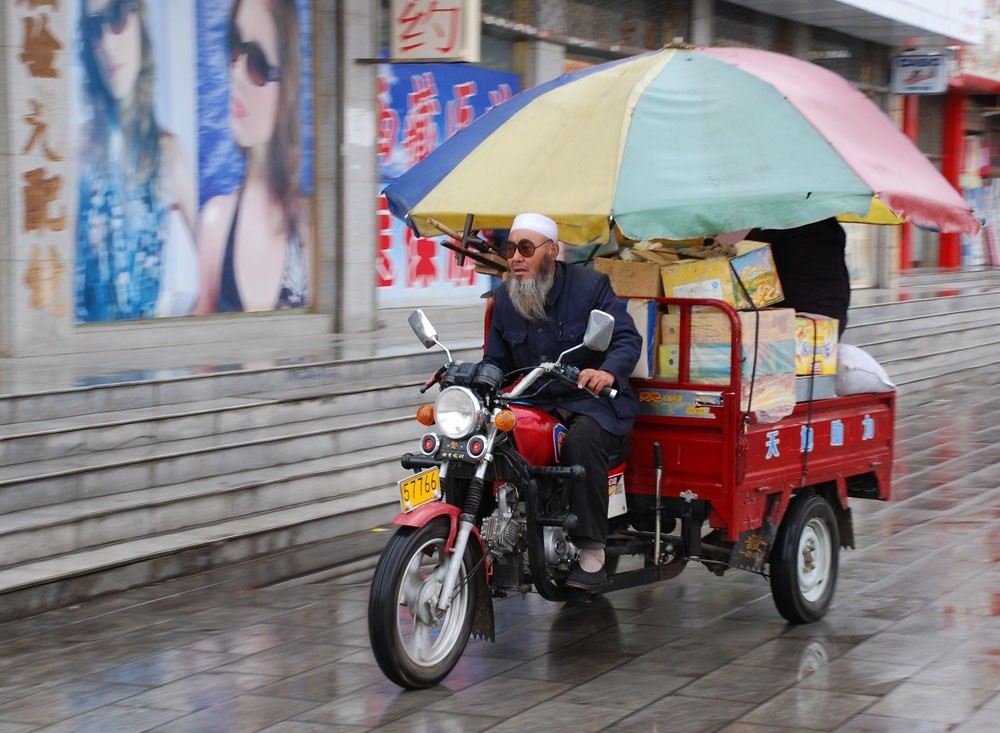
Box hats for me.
[510,214,559,244]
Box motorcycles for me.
[365,292,988,695]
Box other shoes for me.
[567,559,607,590]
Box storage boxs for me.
[593,237,840,410]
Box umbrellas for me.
[380,38,983,252]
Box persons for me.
[424,214,642,591]
[703,214,851,341]
[197,0,317,316]
[70,1,195,322]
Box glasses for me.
[228,25,285,87]
[505,238,553,258]
[86,0,138,47]
